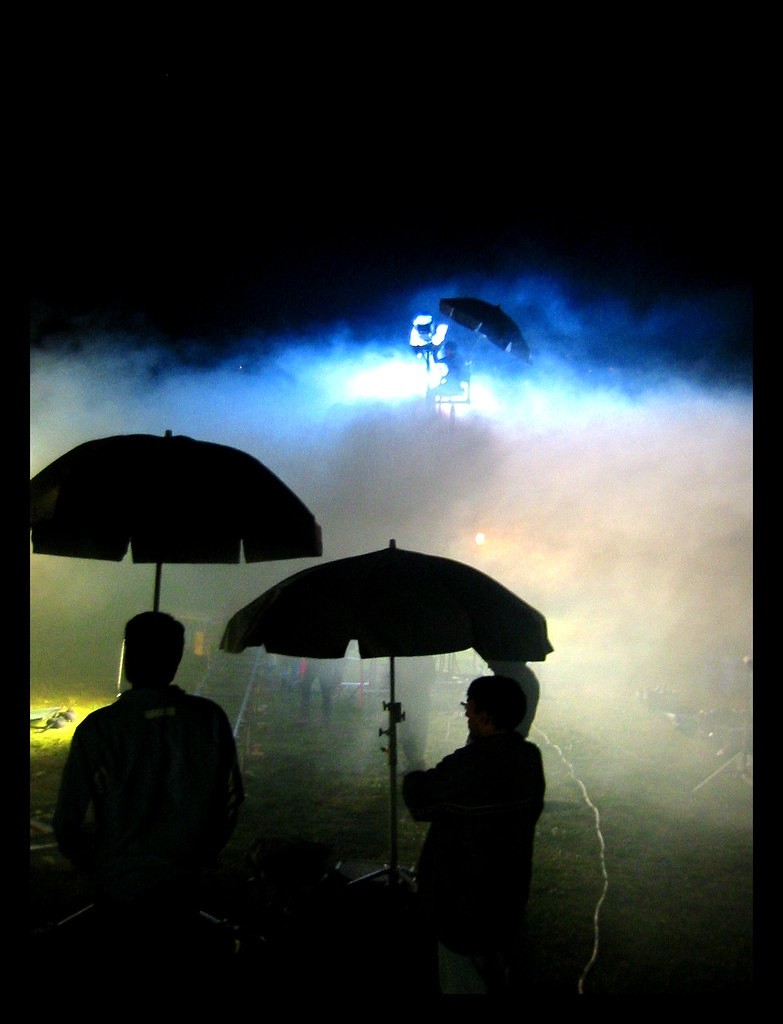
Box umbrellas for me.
[438,298,534,366]
[216,538,552,888]
[28,428,323,612]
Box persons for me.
[51,612,243,1024]
[296,656,335,735]
[487,656,541,739]
[400,677,545,1024]
[425,342,470,412]
[397,656,435,778]
